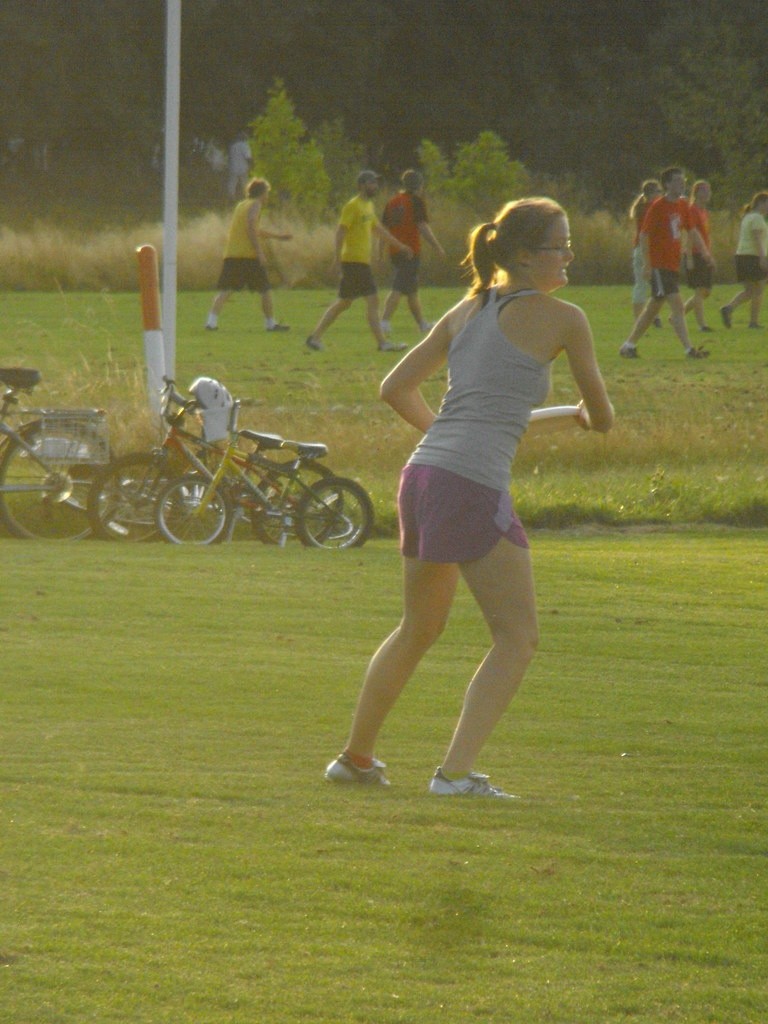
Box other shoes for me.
[700,325,712,332]
[654,317,662,328]
[688,348,710,359]
[419,321,438,331]
[377,342,408,351]
[266,324,289,332]
[380,322,392,337]
[306,337,321,352]
[719,305,732,328]
[205,324,219,331]
[619,344,639,359]
[748,321,763,329]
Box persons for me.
[376,170,444,334]
[305,171,414,352]
[720,192,768,329]
[205,179,292,332]
[323,197,618,799]
[615,168,710,362]
[227,131,252,200]
[629,179,663,336]
[668,181,715,332]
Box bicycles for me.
[0,365,117,544]
[85,373,344,548]
[154,396,375,550]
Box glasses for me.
[528,238,572,252]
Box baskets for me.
[40,415,110,466]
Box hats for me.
[358,172,382,184]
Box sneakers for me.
[430,766,510,797]
[324,753,391,787]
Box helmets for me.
[188,378,233,410]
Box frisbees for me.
[521,405,581,429]
